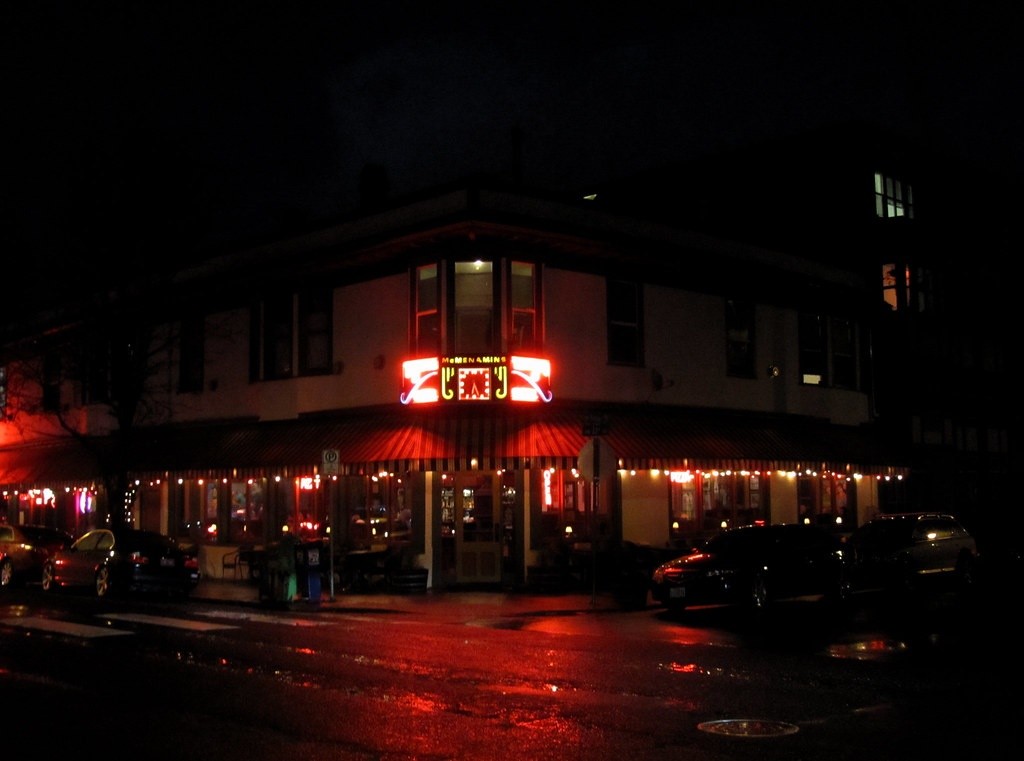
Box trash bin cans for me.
[259,551,273,601]
[273,543,298,602]
[295,540,323,602]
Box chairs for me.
[222,543,254,582]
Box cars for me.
[42,527,202,607]
[0,523,74,593]
[649,522,871,617]
[852,512,980,603]
